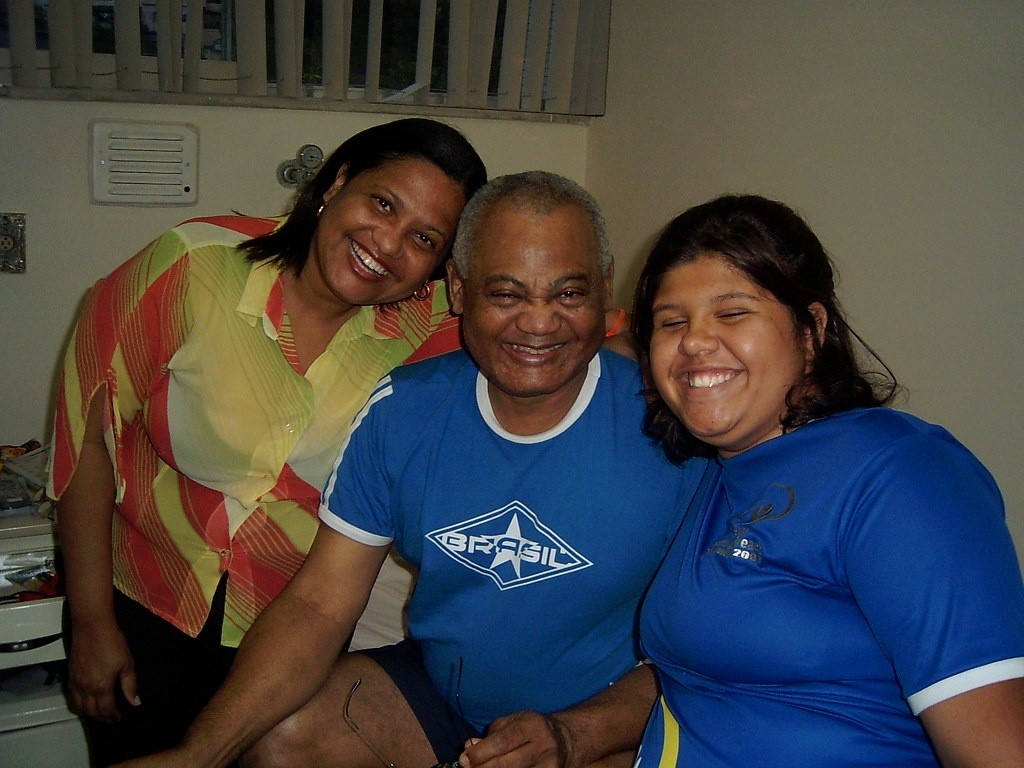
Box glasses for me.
[342,657,474,768]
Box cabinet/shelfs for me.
[0,513,90,768]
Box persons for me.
[41,118,1024,768]
